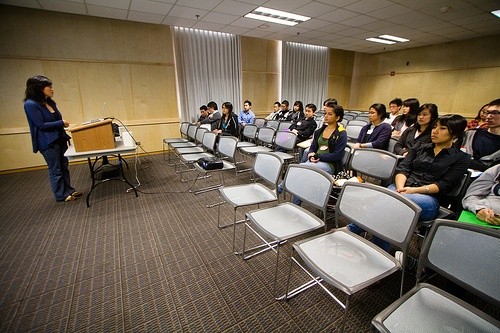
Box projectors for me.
[197,156,224,170]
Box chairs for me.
[162,109,500,333]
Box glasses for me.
[389,106,399,109]
[483,111,500,116]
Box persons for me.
[196,98,500,254]
[23,75,82,202]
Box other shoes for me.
[277,182,284,193]
[71,191,83,197]
[56,194,77,202]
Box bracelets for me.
[425,185,429,194]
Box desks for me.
[63,132,138,208]
